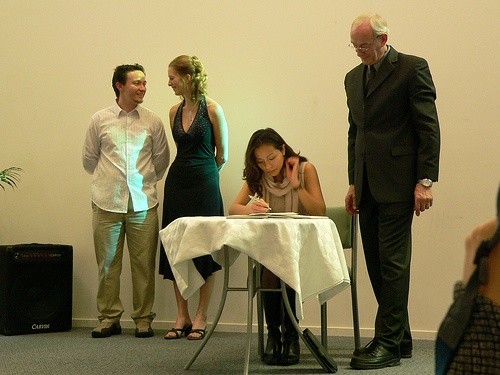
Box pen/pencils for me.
[248,194,272,210]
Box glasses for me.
[348,35,382,51]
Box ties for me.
[364,64,377,95]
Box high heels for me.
[262,318,298,354]
[164,324,193,339]
[188,325,207,340]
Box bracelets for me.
[293,182,303,191]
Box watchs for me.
[418,178,433,187]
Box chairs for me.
[280,205,362,355]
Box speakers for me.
[0,244,72,336]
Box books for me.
[227,212,299,219]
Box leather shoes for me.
[349,340,401,369]
[352,339,412,357]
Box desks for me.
[159,214,351,375]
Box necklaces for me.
[189,101,198,121]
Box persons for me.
[83,64,170,337]
[230,128,326,366]
[158,55,228,340]
[446,187,500,375]
[344,14,440,370]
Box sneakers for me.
[91,321,122,337]
[136,321,154,337]
